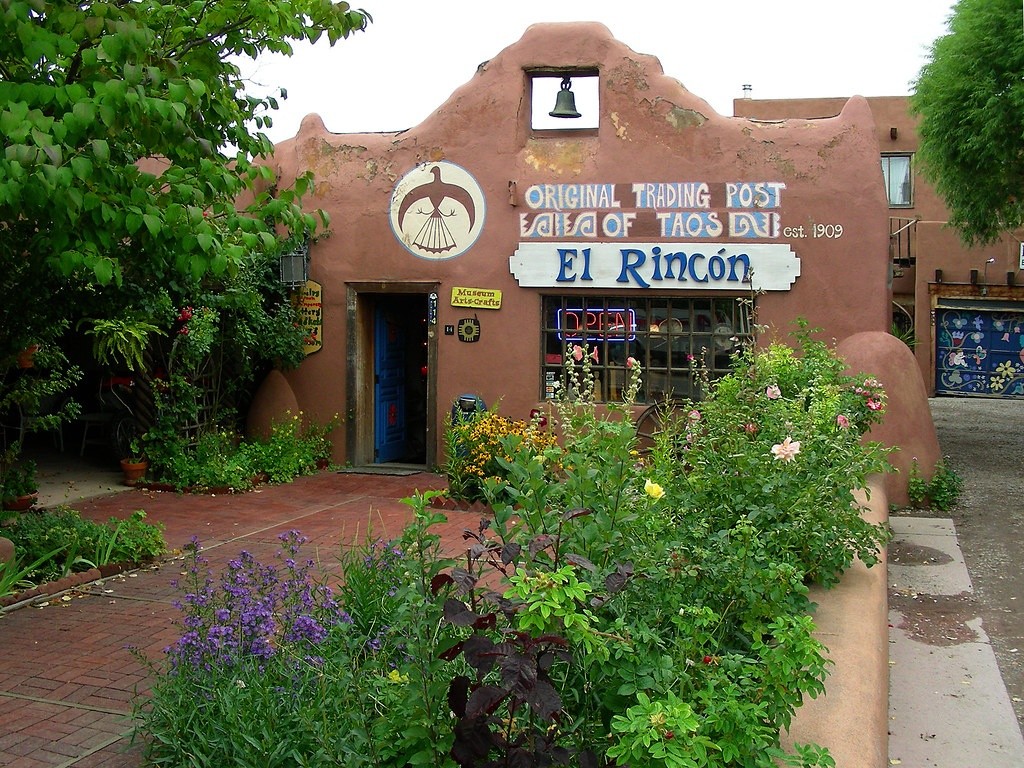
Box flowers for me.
[127,431,147,464]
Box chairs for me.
[18,405,65,453]
[78,376,116,457]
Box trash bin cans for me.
[448,394,487,495]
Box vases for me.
[120,458,149,486]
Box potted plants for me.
[0,457,41,513]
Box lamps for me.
[279,229,311,291]
[984,258,995,283]
